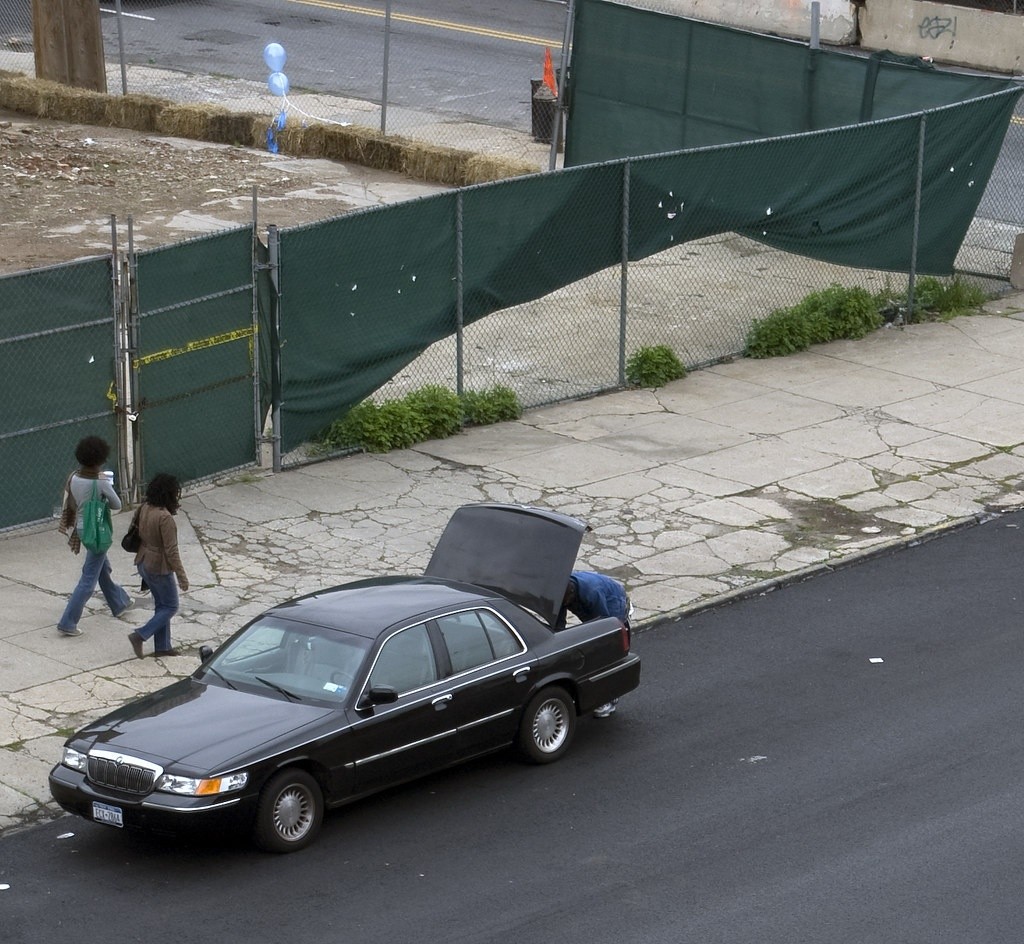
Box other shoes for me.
[155,647,181,657]
[128,632,144,660]
[115,598,135,617]
[57,627,83,636]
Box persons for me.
[128,472,182,659]
[54,435,136,636]
[556,571,633,717]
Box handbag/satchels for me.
[79,480,113,554]
[141,579,150,591]
[121,502,146,553]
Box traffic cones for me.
[543,47,559,98]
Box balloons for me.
[268,72,289,96]
[265,43,287,72]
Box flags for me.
[543,47,558,97]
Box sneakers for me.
[593,702,616,718]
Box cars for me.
[49,502,640,855]
